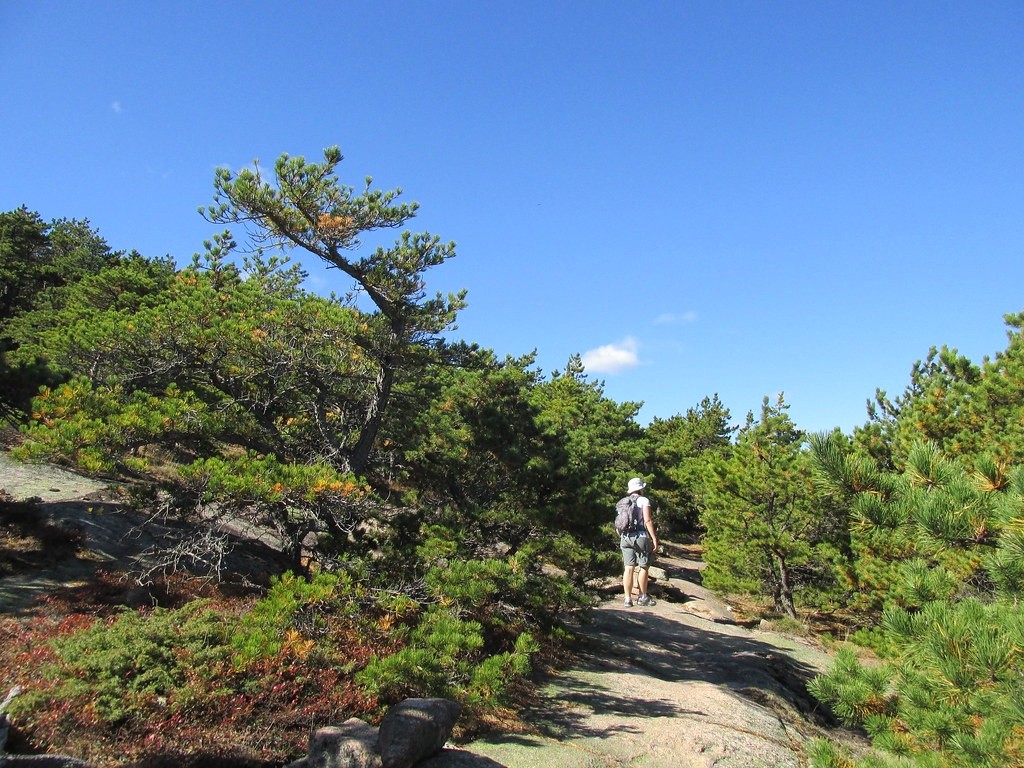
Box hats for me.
[626,477,648,494]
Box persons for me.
[616,478,658,607]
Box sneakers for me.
[637,594,656,606]
[624,597,633,606]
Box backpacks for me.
[617,494,645,535]
